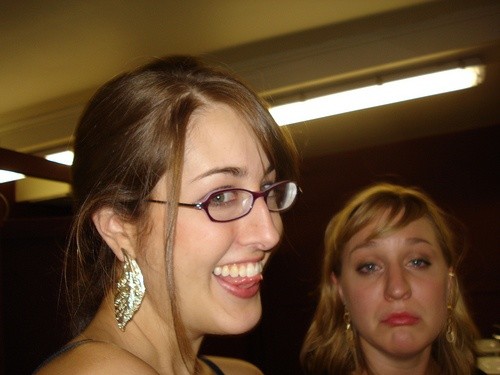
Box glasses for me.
[141,178,299,224]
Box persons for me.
[299,183,490,375]
[34,53,303,374]
